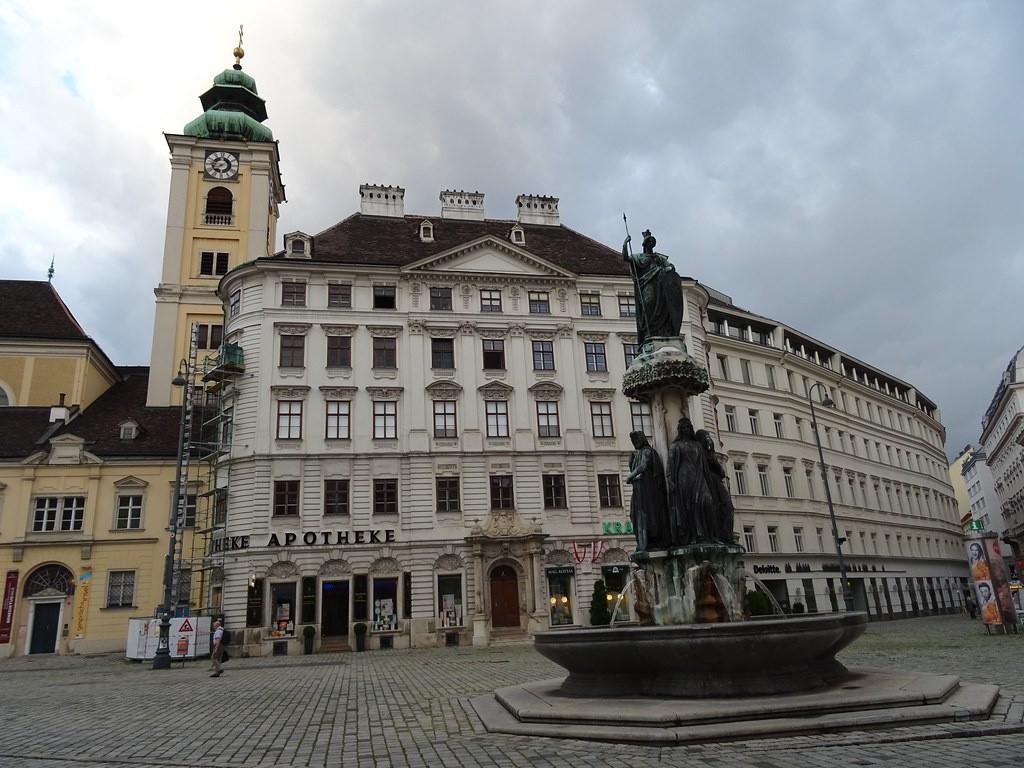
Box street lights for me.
[150,355,189,670]
[808,382,854,611]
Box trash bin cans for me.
[176,636,189,656]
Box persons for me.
[978,581,997,607]
[209,620,227,678]
[621,228,684,348]
[625,429,672,554]
[666,417,735,544]
[969,542,987,569]
[966,595,978,620]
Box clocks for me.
[204,151,239,179]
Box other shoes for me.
[218,670,223,675]
[210,673,219,677]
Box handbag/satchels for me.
[222,652,230,663]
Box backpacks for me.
[217,628,231,646]
